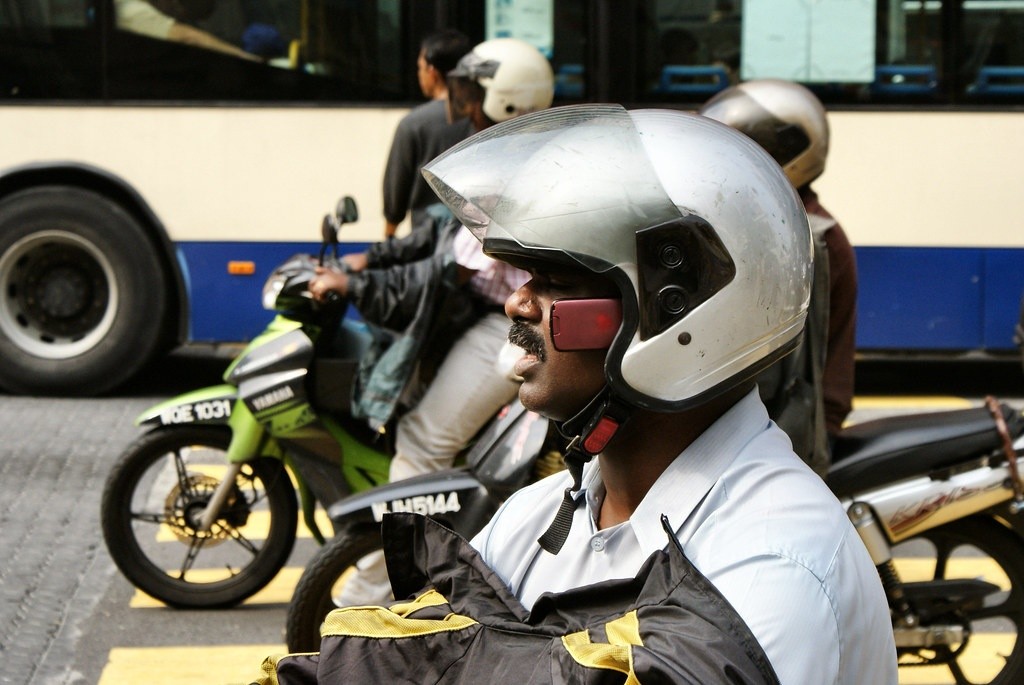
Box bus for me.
[0,0,1024,399]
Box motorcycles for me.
[98,194,563,612]
[281,404,1024,685]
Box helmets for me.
[448,38,555,122]
[481,103,815,412]
[696,80,829,187]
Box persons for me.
[382,31,475,238]
[254,102,900,685]
[698,78,857,465]
[310,38,556,484]
